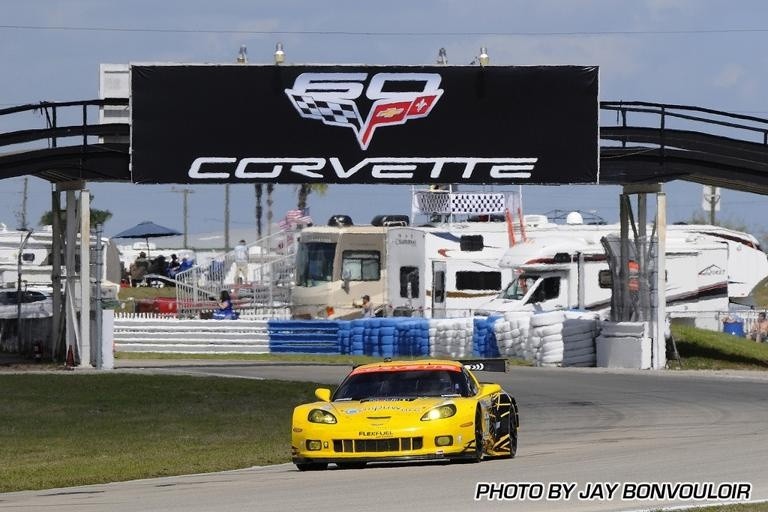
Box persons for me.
[128,253,188,288]
[745,311,768,342]
[233,239,250,284]
[352,294,376,318]
[216,289,233,311]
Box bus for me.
[292,226,385,321]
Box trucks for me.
[134,274,266,315]
[473,239,729,313]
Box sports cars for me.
[289,359,520,470]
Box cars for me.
[0,289,66,319]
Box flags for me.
[280,218,292,230]
[288,206,312,224]
[279,232,294,248]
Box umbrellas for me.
[110,219,184,259]
